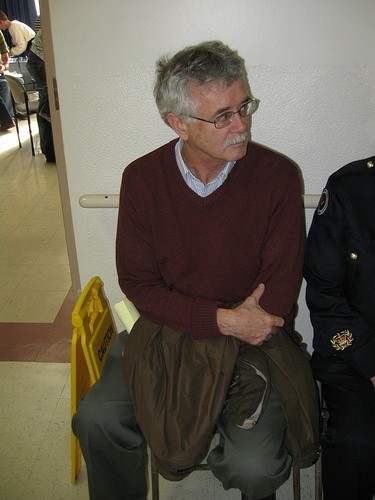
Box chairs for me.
[5,74,40,156]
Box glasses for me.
[180,97,261,130]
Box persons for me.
[71,39,305,500]
[0,30,15,130]
[27,29,55,161]
[0,10,36,58]
[305,155,375,500]
